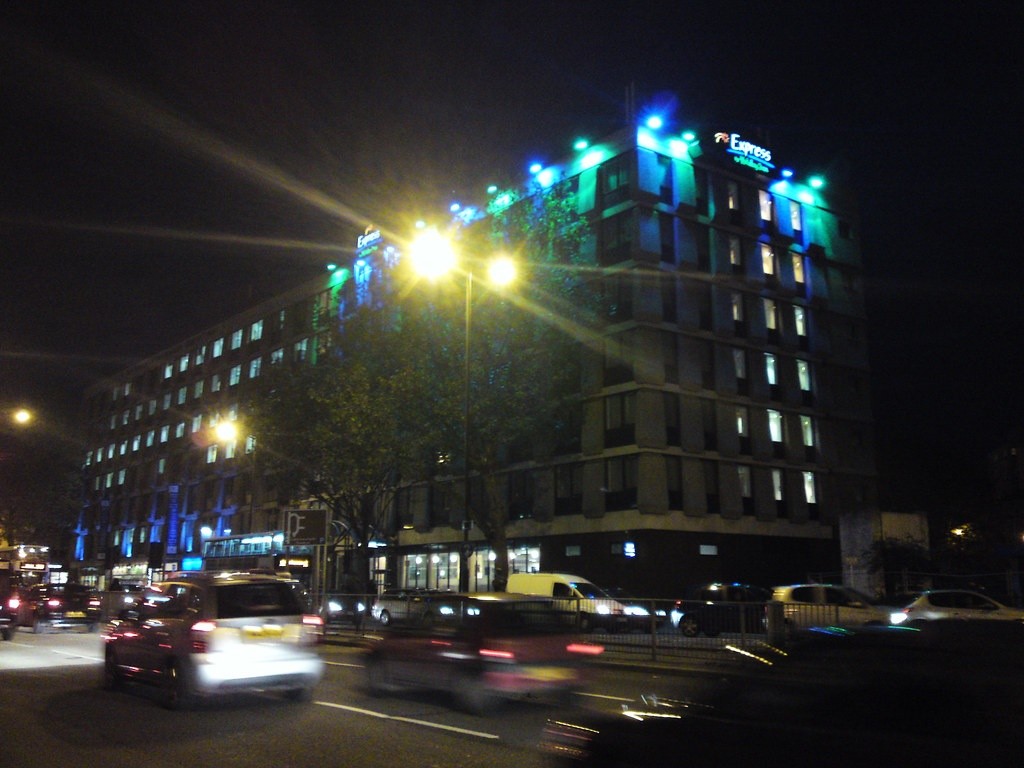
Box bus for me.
[0,544,51,604]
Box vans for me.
[502,573,624,639]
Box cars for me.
[10,583,103,634]
[358,592,595,718]
[772,583,899,629]
[599,584,666,634]
[298,588,480,632]
[884,587,1022,625]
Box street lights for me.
[408,228,519,630]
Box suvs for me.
[671,578,772,637]
[98,570,326,710]
